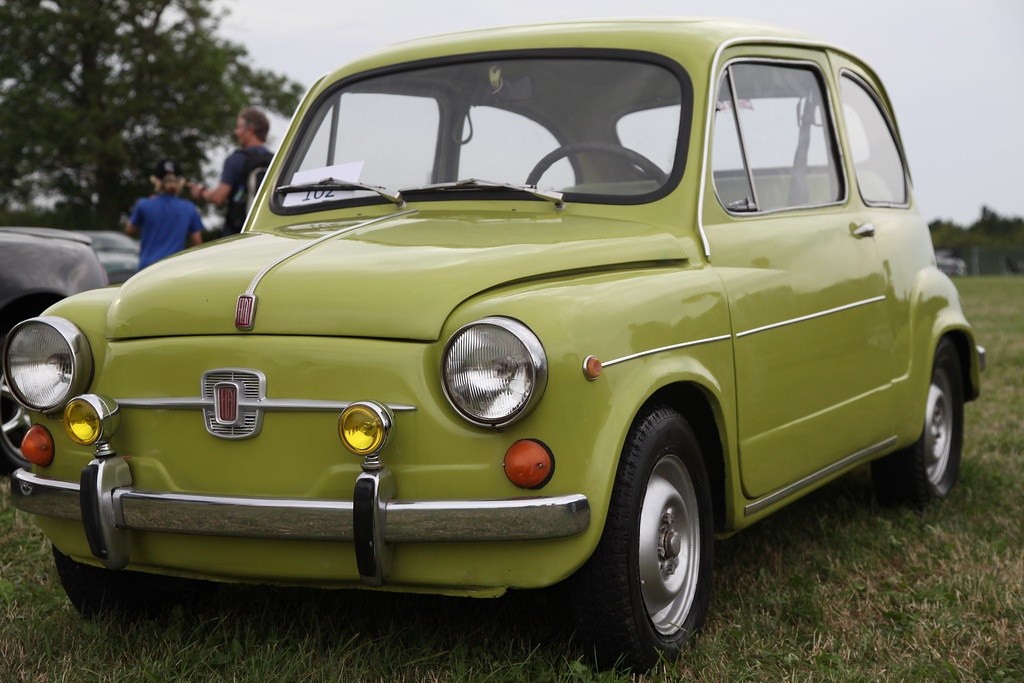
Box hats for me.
[156,160,181,179]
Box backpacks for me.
[230,150,270,222]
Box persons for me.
[187,109,275,236]
[124,160,206,271]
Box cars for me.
[0,221,141,473]
[0,16,985,670]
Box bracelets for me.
[199,186,206,197]
